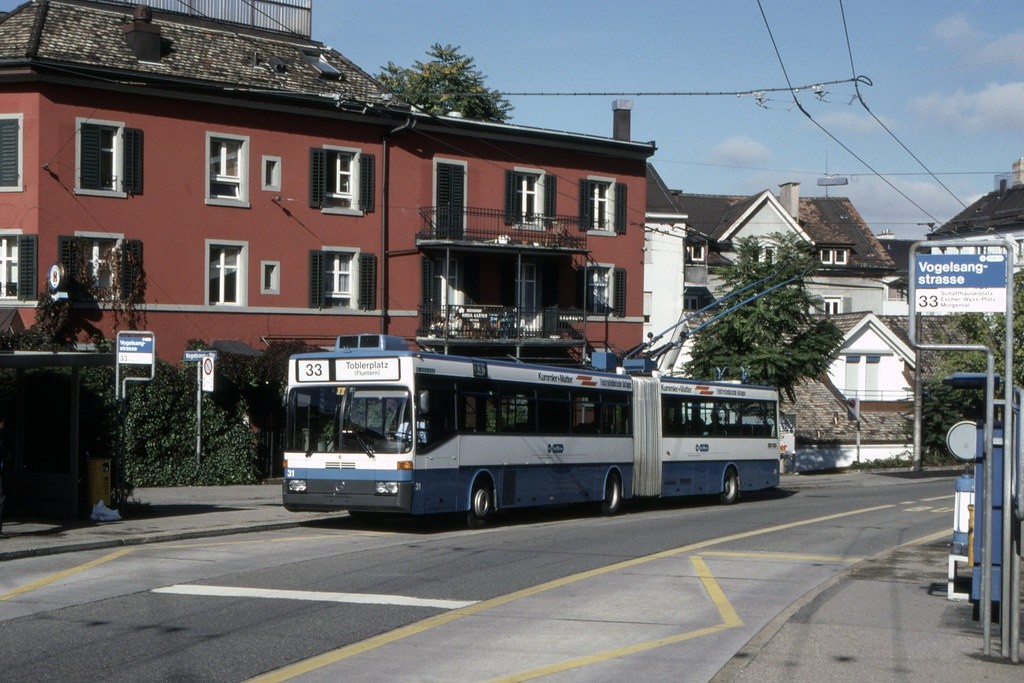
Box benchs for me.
[947,540,971,600]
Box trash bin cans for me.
[86,456,112,507]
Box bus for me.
[281,272,799,529]
